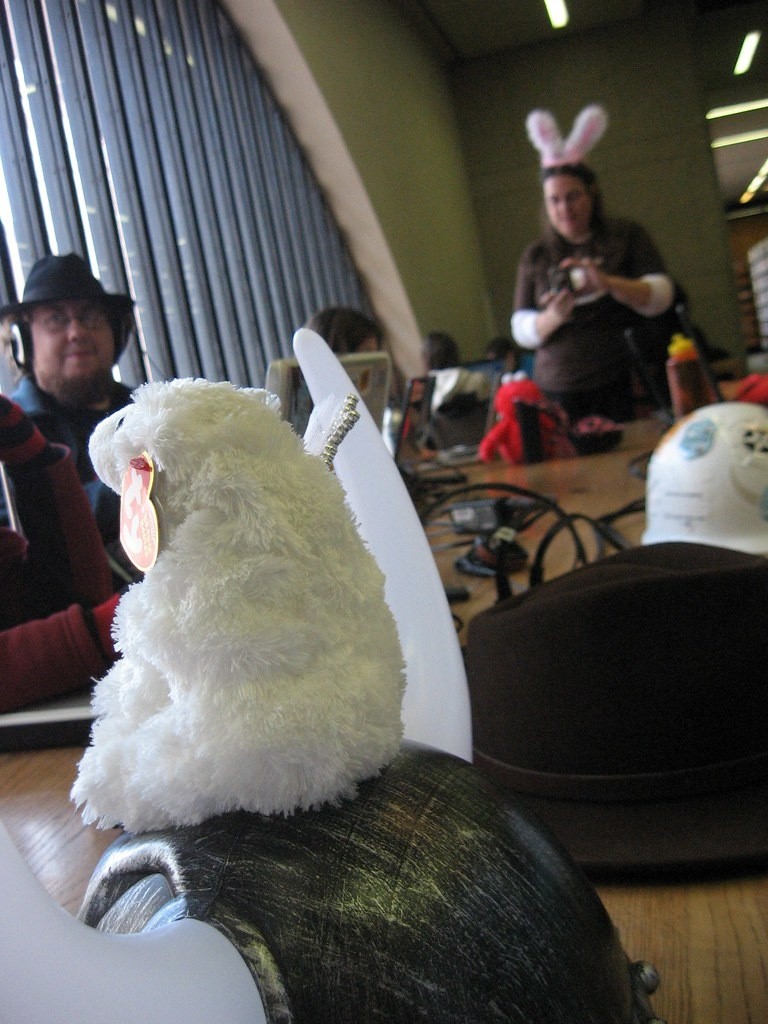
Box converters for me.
[449,494,511,534]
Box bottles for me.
[667,334,718,425]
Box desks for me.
[0,417,768,1023]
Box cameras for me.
[547,265,573,297]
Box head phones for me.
[8,309,126,367]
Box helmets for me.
[640,400,768,559]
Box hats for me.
[1,254,134,362]
[466,541,768,882]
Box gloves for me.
[0,395,46,467]
[85,581,141,669]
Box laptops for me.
[267,350,506,477]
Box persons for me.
[308,307,386,356]
[511,163,677,423]
[0,252,145,585]
[0,397,125,715]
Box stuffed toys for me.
[74,377,408,830]
